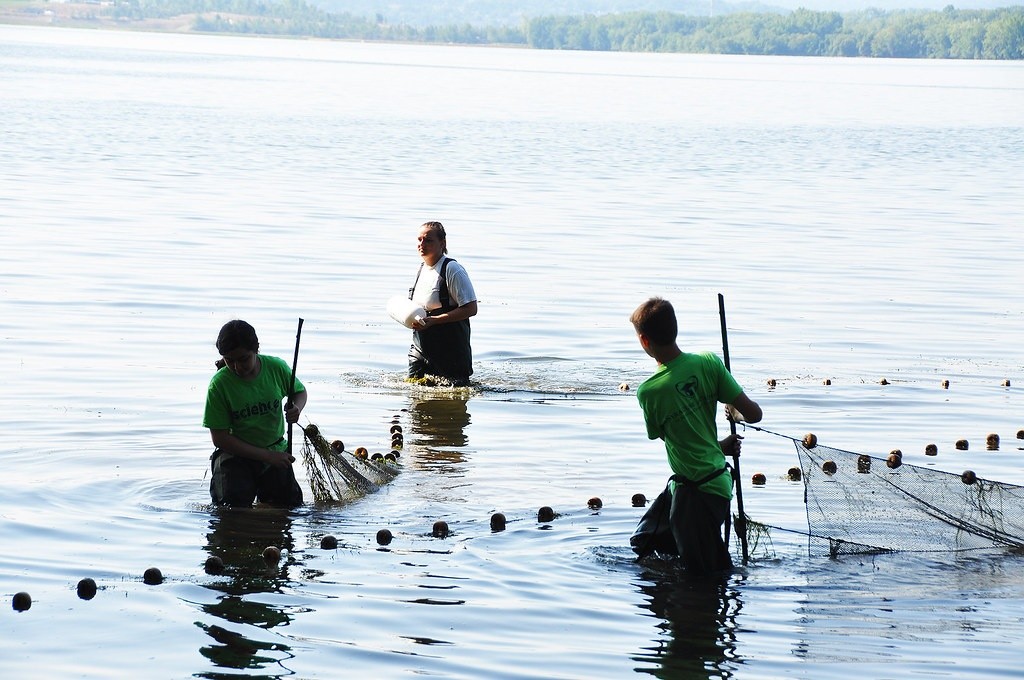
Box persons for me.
[629,300,762,574]
[406,221,477,379]
[201,320,307,508]
